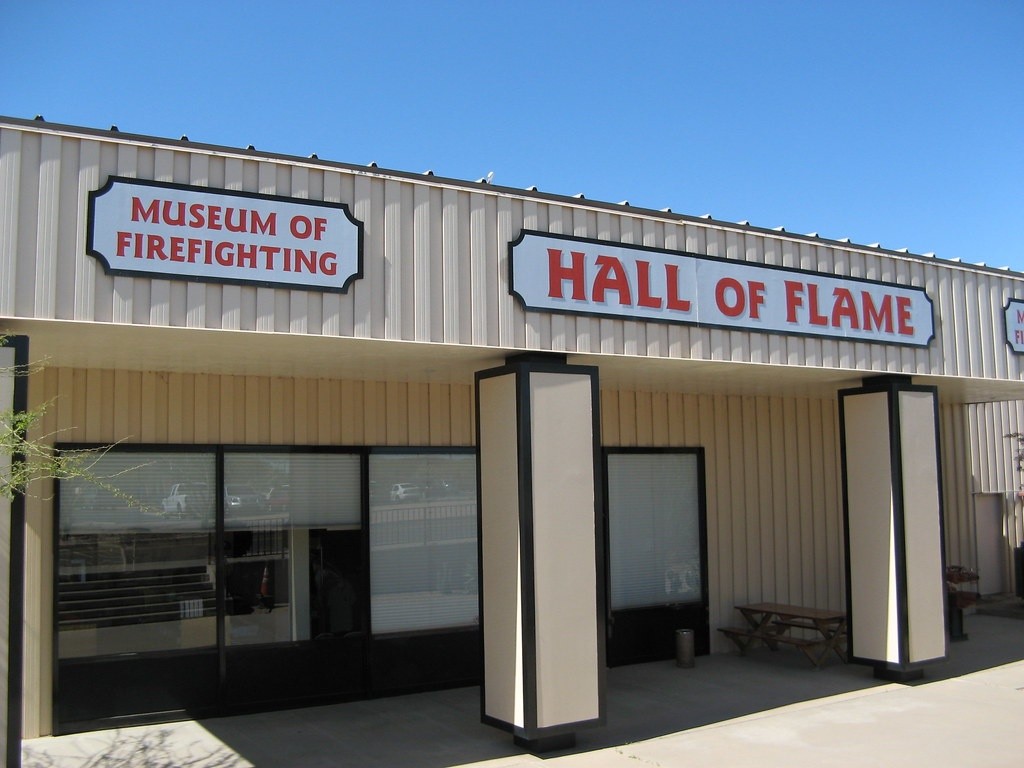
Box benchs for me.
[771,620,848,664]
[716,627,824,671]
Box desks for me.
[733,602,846,672]
